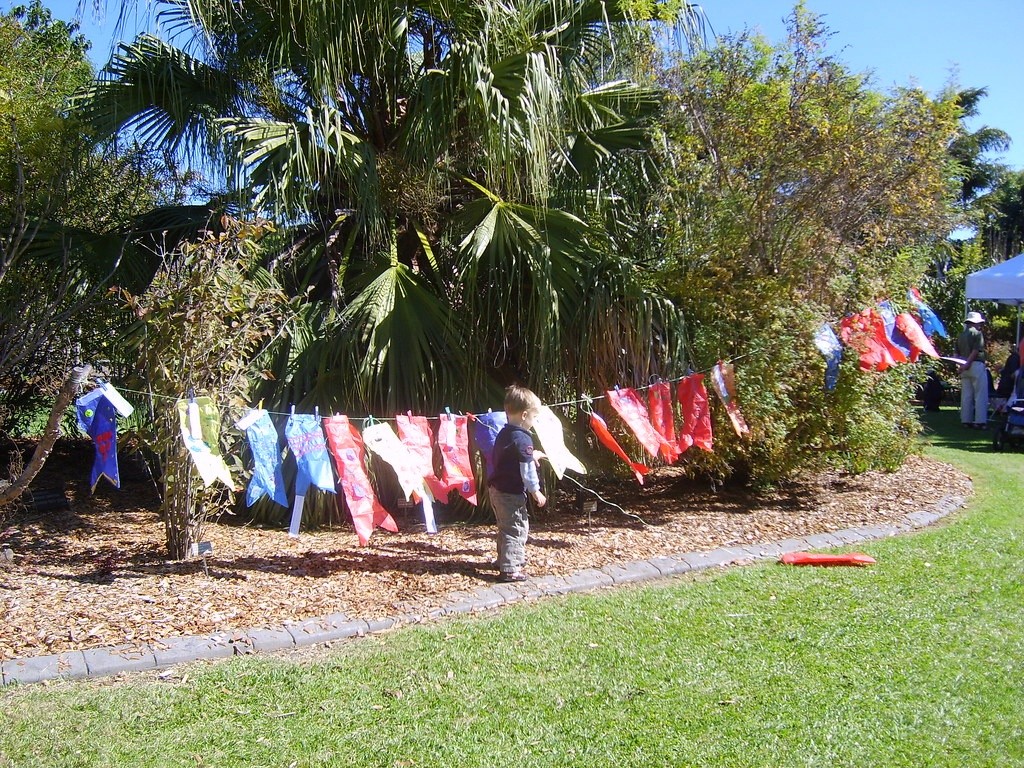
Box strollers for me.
[992,381,1024,452]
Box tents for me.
[964,253,1024,353]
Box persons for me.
[920,362,944,411]
[487,386,546,582]
[995,353,1020,410]
[956,312,992,430]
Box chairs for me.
[986,369,1007,421]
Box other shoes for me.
[495,558,526,567]
[963,422,974,428]
[973,423,991,430]
[499,571,528,581]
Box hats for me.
[964,312,985,324]
